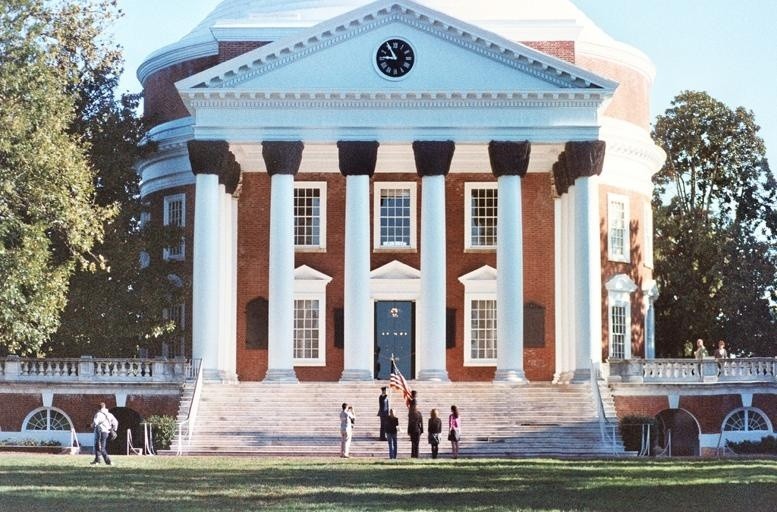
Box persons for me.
[448,405,461,459]
[406,390,417,417]
[715,340,728,376]
[427,408,442,458]
[407,399,424,458]
[377,386,390,441]
[694,338,709,376]
[87,402,120,464]
[385,409,399,459]
[340,402,355,459]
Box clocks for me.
[372,36,417,82]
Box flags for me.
[389,360,412,399]
